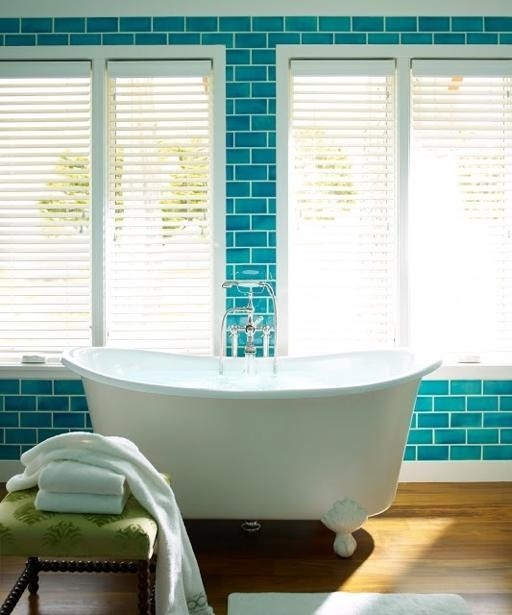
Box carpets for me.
[226,589,475,615]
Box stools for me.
[1,473,173,614]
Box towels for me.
[32,490,129,516]
[37,459,129,496]
[6,428,215,615]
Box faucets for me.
[230,315,270,358]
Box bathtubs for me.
[60,347,443,560]
